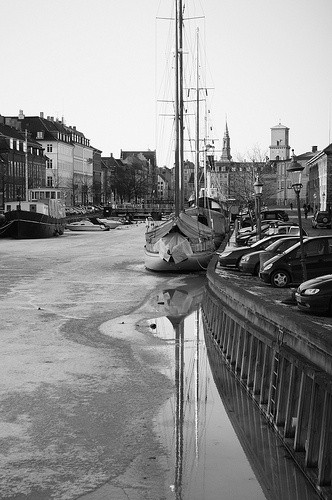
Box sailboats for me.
[141,0,231,272]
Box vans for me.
[256,234,332,288]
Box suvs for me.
[241,209,289,228]
[311,211,332,230]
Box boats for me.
[66,220,106,231]
[0,188,68,241]
[96,217,122,229]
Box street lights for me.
[252,175,264,239]
[285,148,309,282]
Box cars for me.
[217,233,309,268]
[236,219,309,247]
[293,274,332,317]
[238,236,312,275]
